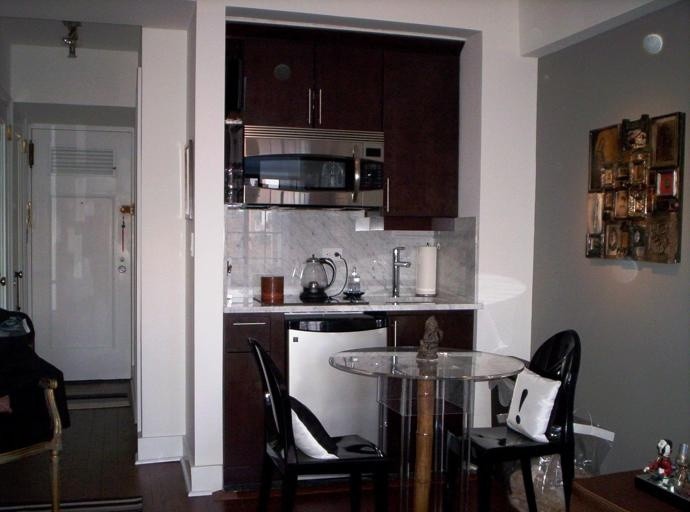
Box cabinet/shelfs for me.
[239,41,384,134]
[387,311,474,452]
[384,54,457,217]
[225,316,285,484]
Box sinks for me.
[385,297,439,305]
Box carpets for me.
[0,493,143,511]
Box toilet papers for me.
[415,247,438,295]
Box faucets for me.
[392,246,411,295]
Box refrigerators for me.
[285,313,389,459]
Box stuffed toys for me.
[643,438,673,480]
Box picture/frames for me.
[651,114,683,170]
[585,124,622,193]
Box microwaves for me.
[223,124,387,213]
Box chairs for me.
[460,328,579,512]
[245,337,388,512]
[0,310,68,512]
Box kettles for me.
[298,253,337,303]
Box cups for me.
[260,275,285,306]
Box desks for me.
[329,346,525,512]
[569,467,687,512]
